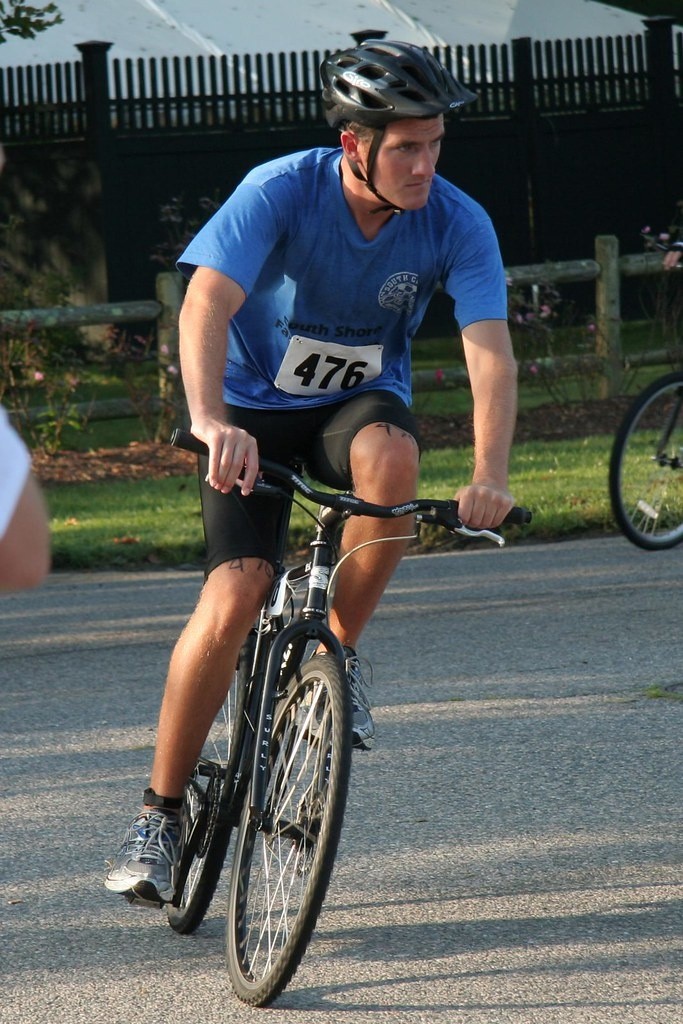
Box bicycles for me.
[609,225,683,552]
[125,426,534,1009]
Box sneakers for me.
[308,645,375,746]
[105,809,184,904]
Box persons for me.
[0,406,50,590]
[105,40,519,905]
[662,235,683,269]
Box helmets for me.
[320,40,478,127]
